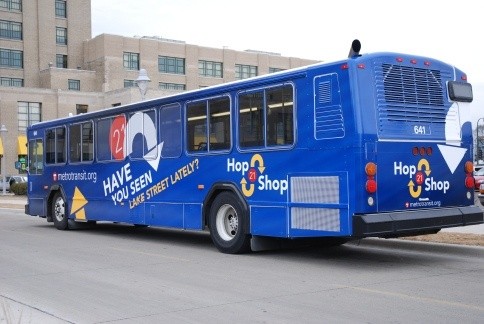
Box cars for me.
[473,167,484,188]
[0,176,27,190]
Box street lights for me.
[0,125,8,194]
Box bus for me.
[25,39,484,254]
